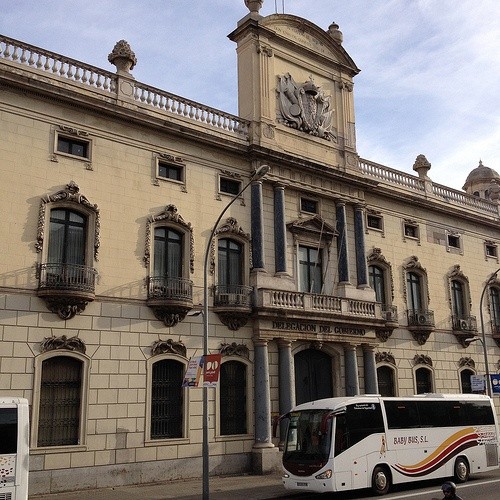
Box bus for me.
[276,392,500,496]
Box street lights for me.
[186,164,271,500]
